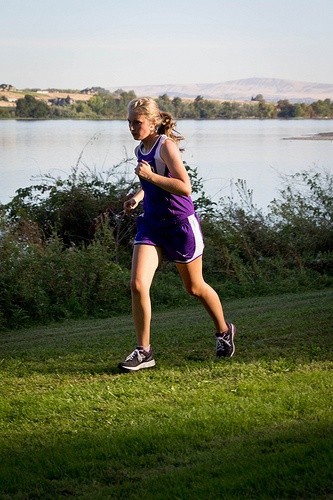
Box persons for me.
[118,97,237,370]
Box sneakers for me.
[215,322,236,358]
[121,346,155,370]
